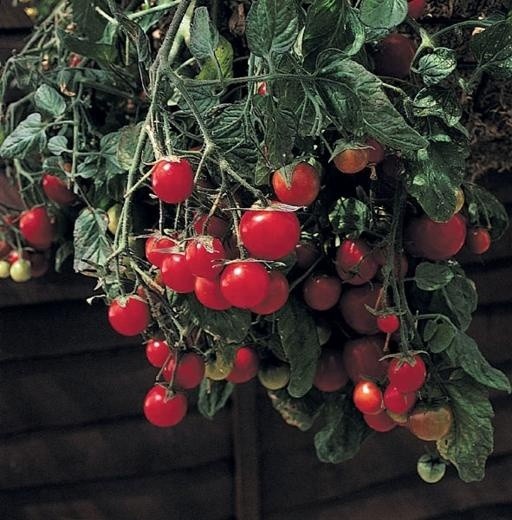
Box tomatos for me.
[373,32,415,78]
[0,84,490,484]
[408,0,425,17]
